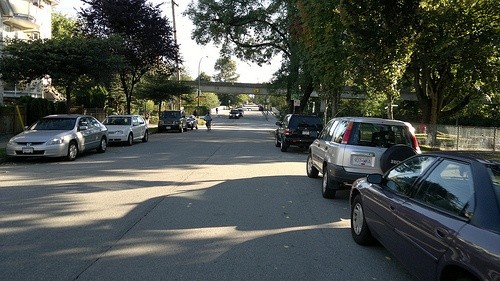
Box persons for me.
[216,108,218,114]
[194,109,198,116]
[204,112,212,126]
[418,117,426,133]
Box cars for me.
[6,114,108,161]
[102,115,148,146]
[348,150,500,281]
[186,115,199,130]
[275,114,324,153]
[229,108,245,119]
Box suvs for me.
[158,110,186,133]
[306,116,423,200]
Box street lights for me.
[197,55,209,106]
